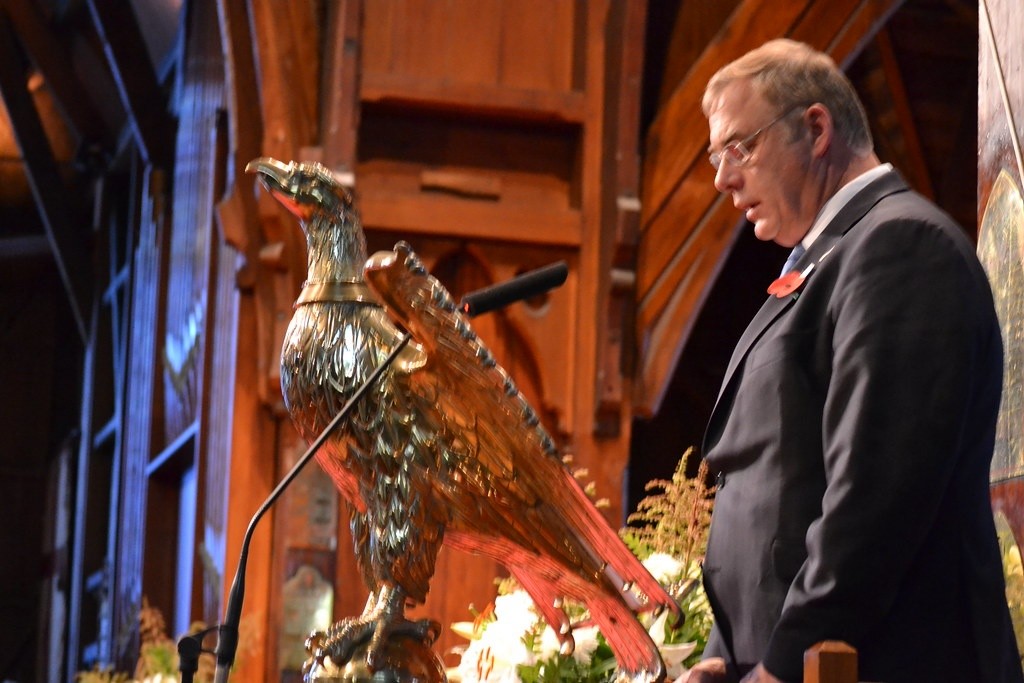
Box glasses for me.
[709,102,800,172]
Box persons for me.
[681,38,1024,682]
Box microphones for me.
[214,259,571,683]
